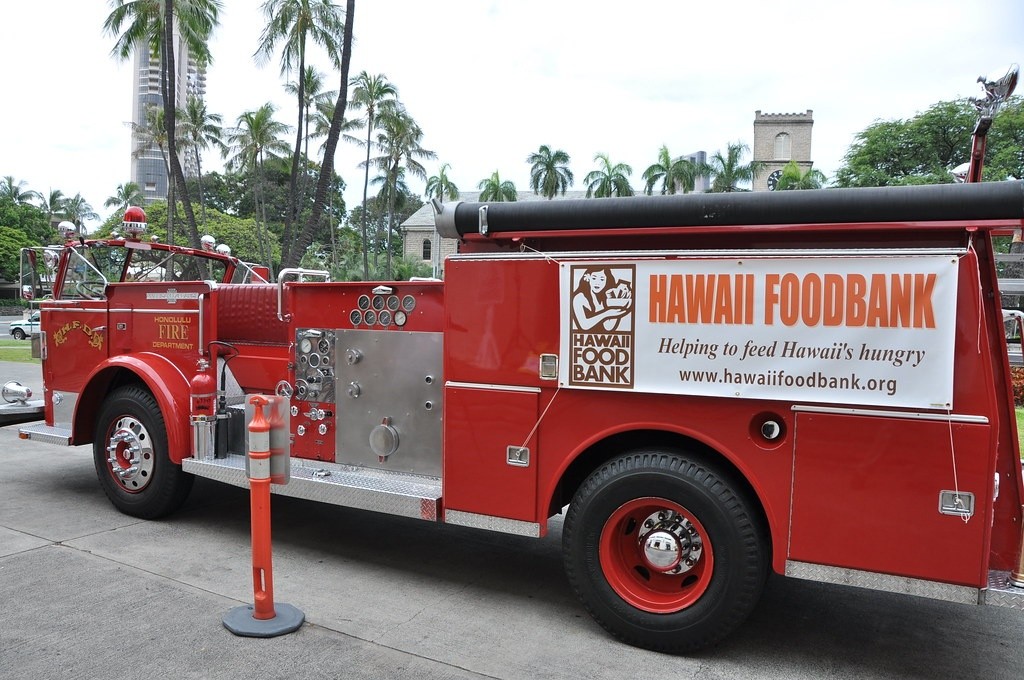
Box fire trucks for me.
[0,63,1024,656]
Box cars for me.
[9,311,41,339]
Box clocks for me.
[768,171,792,191]
